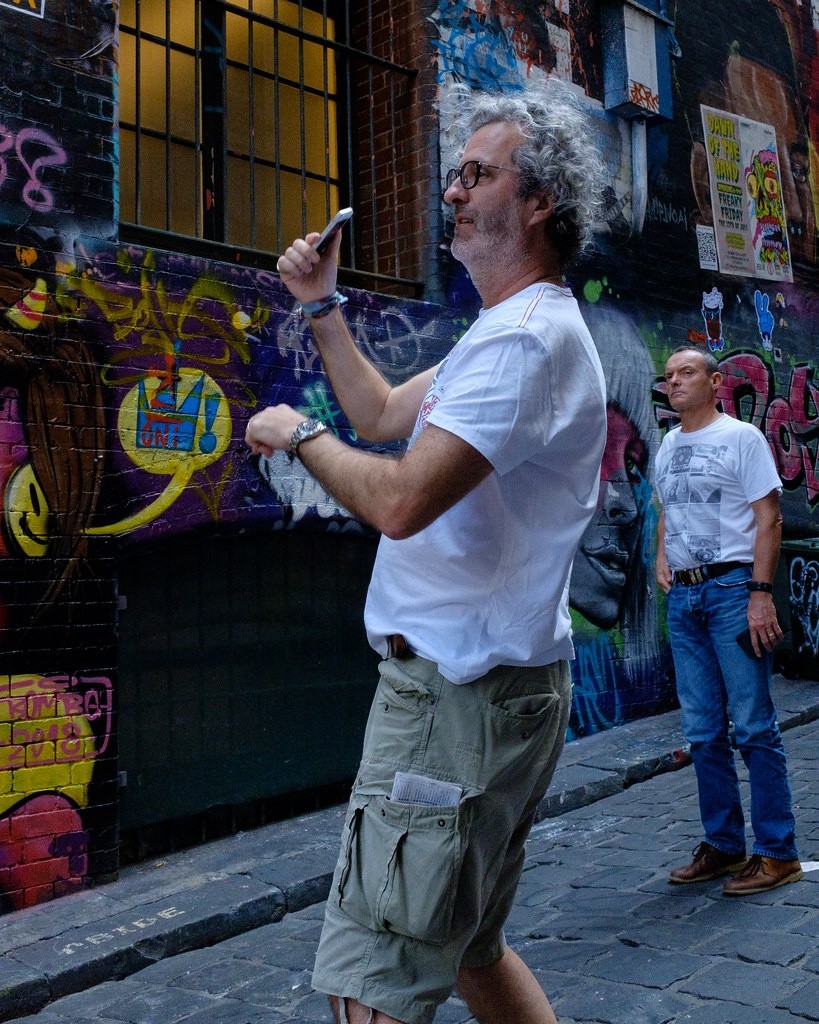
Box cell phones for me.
[312,207,353,256]
[737,627,765,658]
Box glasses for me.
[446,160,519,190]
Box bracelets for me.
[300,289,349,318]
[747,581,775,595]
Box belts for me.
[669,561,754,587]
[387,634,407,658]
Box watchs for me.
[289,418,325,456]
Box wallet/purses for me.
[737,611,790,660]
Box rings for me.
[247,421,251,429]
[768,633,774,637]
[276,263,285,273]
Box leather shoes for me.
[722,853,803,894]
[668,841,747,883]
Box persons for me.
[649,346,805,894]
[241,91,615,1024]
